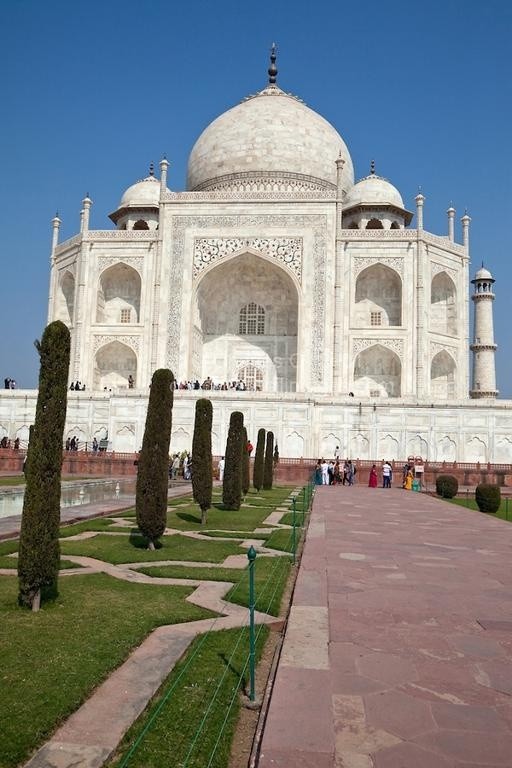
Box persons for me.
[66,436,80,451]
[369,465,378,488]
[69,381,85,391]
[93,438,98,451]
[10,380,15,389]
[382,461,392,488]
[403,465,408,488]
[334,446,339,460]
[388,463,392,488]
[217,457,225,481]
[247,440,253,456]
[174,377,245,390]
[0,437,20,449]
[405,467,413,490]
[5,377,11,389]
[128,375,134,388]
[169,455,192,480]
[315,460,355,486]
